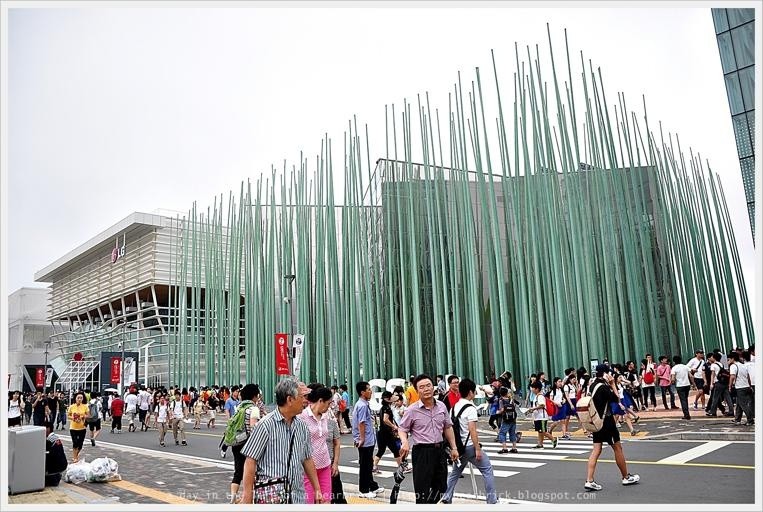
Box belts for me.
[418,443,444,449]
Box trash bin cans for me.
[7,424,46,495]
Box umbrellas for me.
[388,453,410,504]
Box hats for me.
[696,349,703,353]
[381,391,399,403]
[490,382,500,389]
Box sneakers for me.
[497,449,508,454]
[508,449,517,453]
[615,405,679,436]
[621,473,640,486]
[221,449,226,458]
[494,428,570,450]
[584,480,602,490]
[372,467,381,475]
[373,488,385,493]
[358,491,377,498]
[402,467,412,474]
[56,423,215,463]
[682,402,755,426]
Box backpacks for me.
[575,382,608,433]
[224,403,254,447]
[544,388,556,400]
[498,396,517,424]
[715,363,730,385]
[451,404,481,457]
[535,394,559,418]
[336,394,346,413]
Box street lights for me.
[43,340,50,393]
[283,275,297,375]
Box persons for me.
[304,382,347,504]
[351,381,385,499]
[7,372,477,476]
[241,377,324,504]
[229,383,261,504]
[584,364,640,491]
[397,374,460,504]
[296,387,333,504]
[478,345,755,454]
[440,378,497,504]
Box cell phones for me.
[602,372,609,381]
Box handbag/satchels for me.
[253,476,291,504]
[688,370,695,385]
[644,372,654,384]
[657,375,662,386]
[730,387,738,397]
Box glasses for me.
[450,381,459,384]
